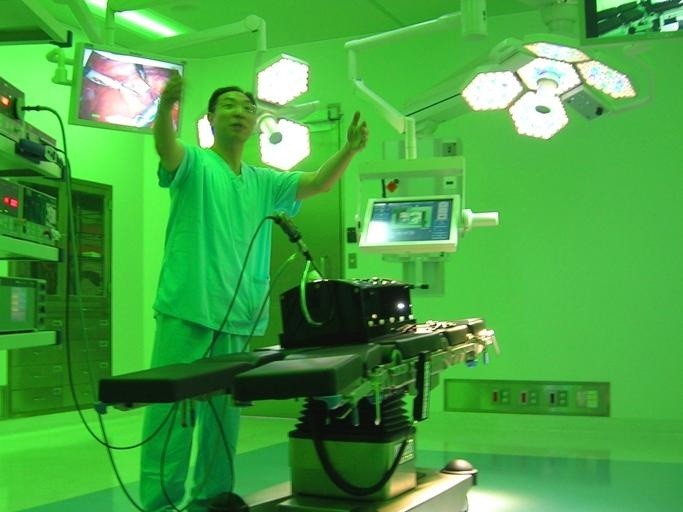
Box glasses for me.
[219,102,256,113]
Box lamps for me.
[458,33,648,140]
[194,51,313,173]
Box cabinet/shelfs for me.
[0,133,66,351]
[10,175,112,416]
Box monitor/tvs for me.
[68,43,188,138]
[359,194,460,254]
[577,0,683,43]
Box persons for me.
[139,71,370,512]
